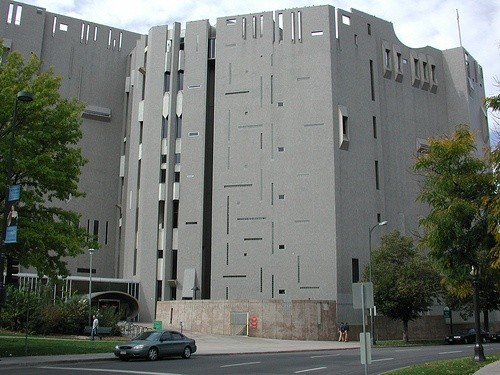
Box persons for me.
[338,322,349,342]
[91,314,102,340]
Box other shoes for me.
[100,336,102,340]
[91,338,94,340]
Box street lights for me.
[469,264,487,362]
[368,221,389,345]
[88,247,95,327]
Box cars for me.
[114,329,197,361]
[445,328,489,345]
[490,331,500,343]
